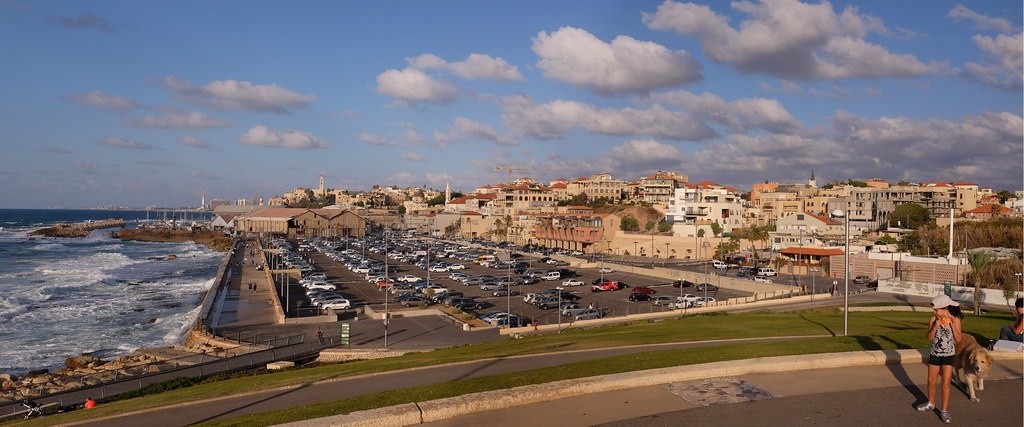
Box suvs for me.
[592,280,620,292]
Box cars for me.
[855,275,871,284]
[599,267,614,274]
[266,227,586,327]
[673,280,694,288]
[575,307,600,321]
[693,297,716,306]
[631,286,657,294]
[677,294,701,303]
[696,283,717,291]
[592,279,609,284]
[754,276,772,284]
[667,299,693,309]
[629,292,651,302]
[651,296,673,306]
[869,280,878,287]
[712,260,760,277]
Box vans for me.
[758,267,778,277]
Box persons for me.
[589,301,600,311]
[998,298,1024,343]
[915,294,965,424]
[85,396,95,408]
[231,242,264,293]
[316,326,324,342]
[305,253,315,265]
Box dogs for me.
[953,333,994,401]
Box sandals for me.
[917,401,936,412]
[940,409,953,424]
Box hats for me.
[929,293,960,309]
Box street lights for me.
[831,208,849,336]
[556,287,564,333]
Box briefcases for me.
[992,339,1024,353]
